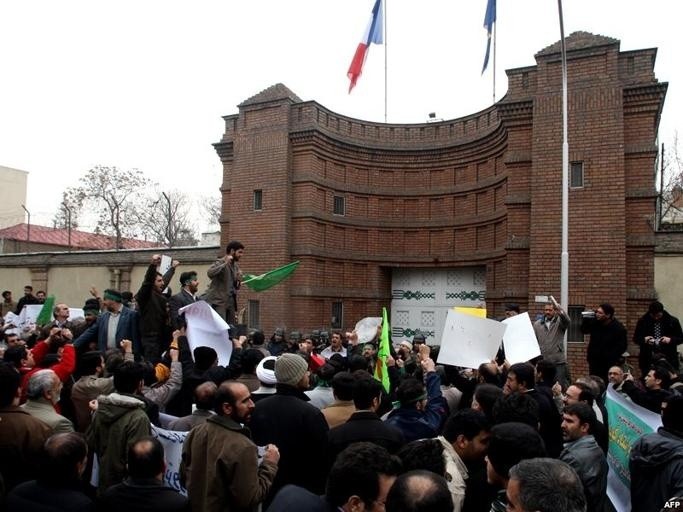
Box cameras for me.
[581,312,595,319]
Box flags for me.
[483,1,498,72]
[345,1,383,97]
[239,261,301,294]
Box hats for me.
[275,354,309,386]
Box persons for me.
[134,255,178,361]
[166,270,198,320]
[197,243,246,319]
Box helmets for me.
[413,333,424,345]
[272,328,331,341]
